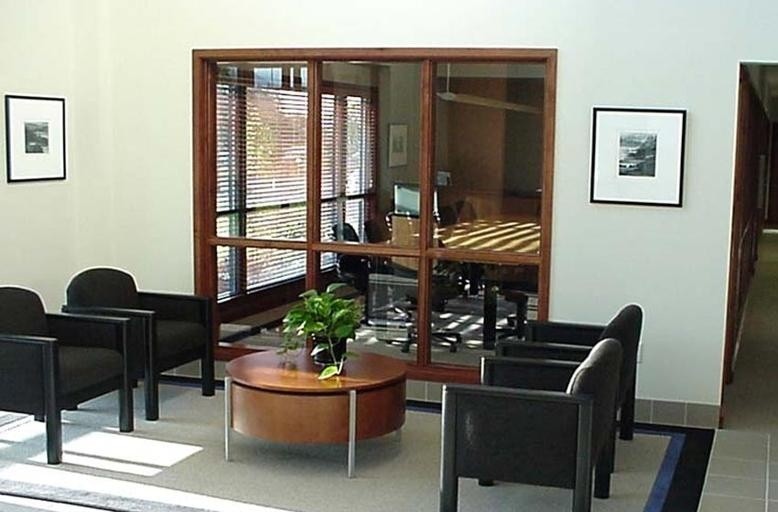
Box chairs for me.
[63,265,214,422]
[438,337,624,511]
[508,304,646,472]
[331,182,541,352]
[0,286,134,465]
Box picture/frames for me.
[590,107,686,208]
[388,124,407,168]
[4,93,66,183]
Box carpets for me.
[0,380,685,512]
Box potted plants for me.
[274,281,365,378]
